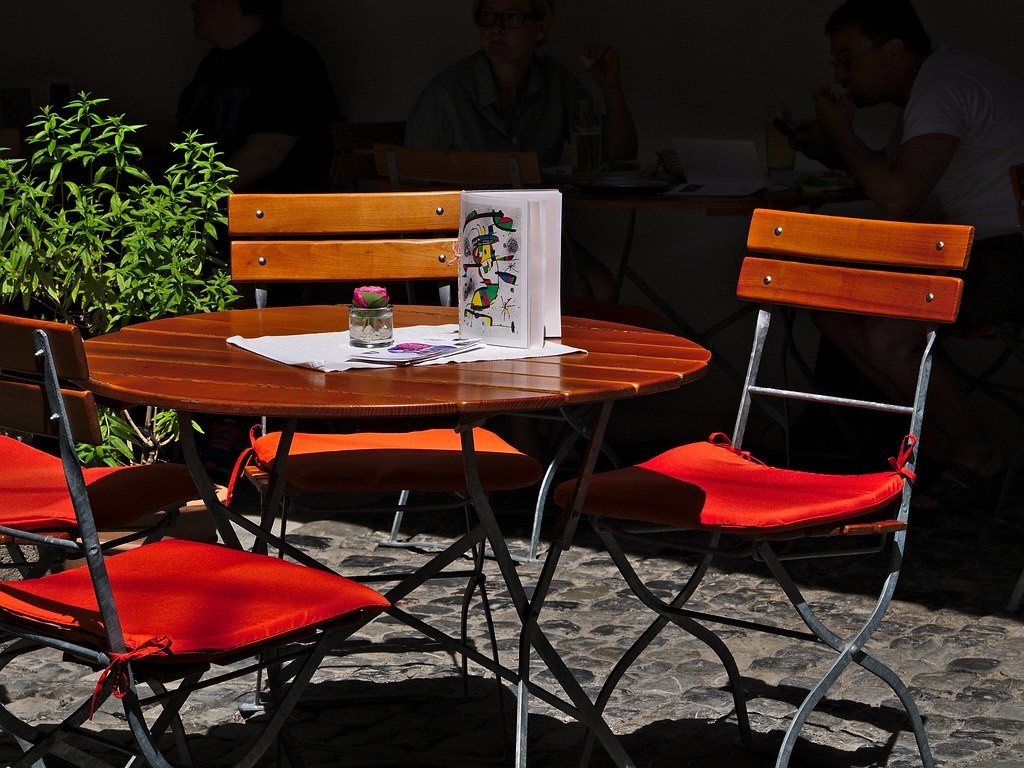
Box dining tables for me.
[69,305,713,768]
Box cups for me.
[348,304,394,348]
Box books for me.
[457,187,562,351]
[346,336,486,367]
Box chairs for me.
[230,193,545,750]
[0,434,215,581]
[553,207,974,768]
[1,315,392,768]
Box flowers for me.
[352,285,390,308]
